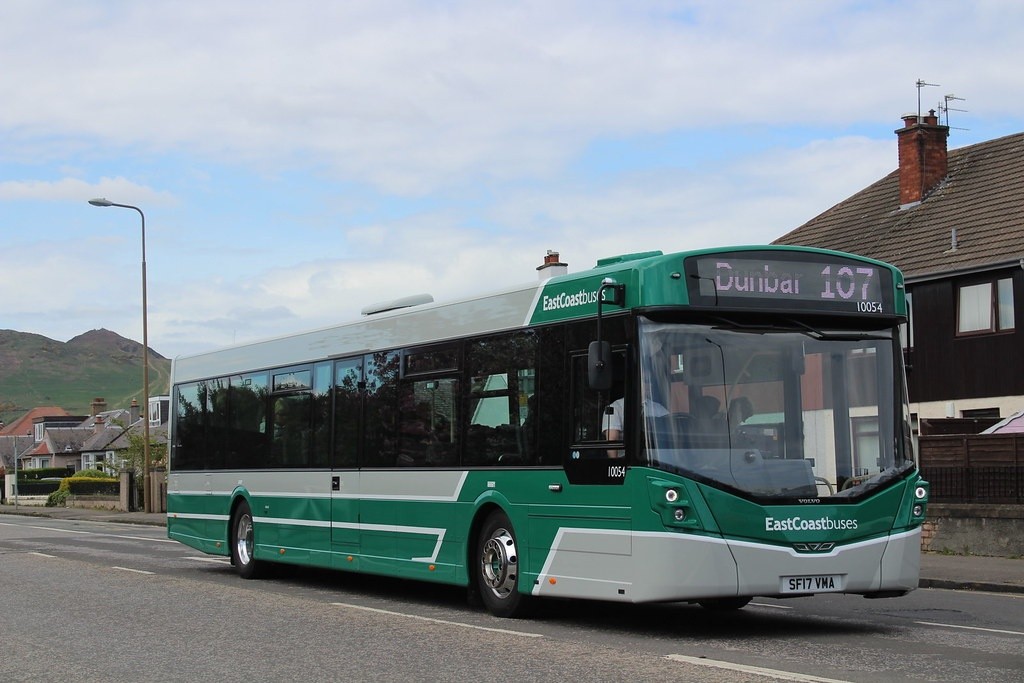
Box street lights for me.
[88,197,152,514]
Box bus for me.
[167,245,929,619]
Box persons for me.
[600,367,672,459]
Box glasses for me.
[642,376,655,385]
